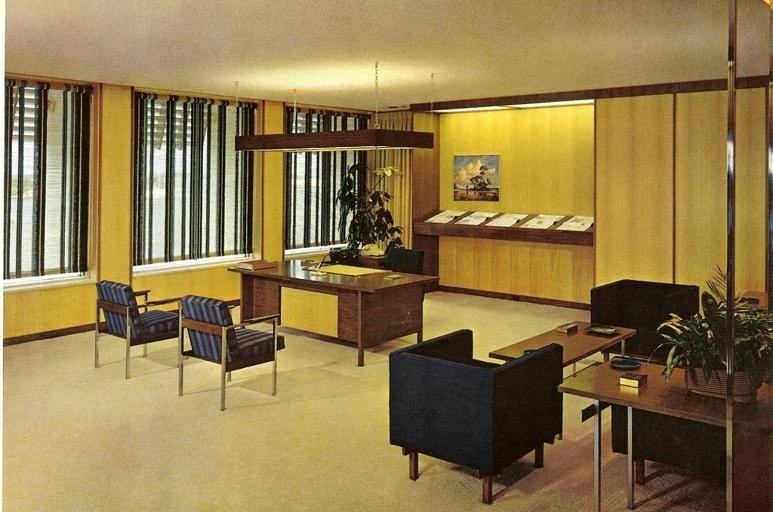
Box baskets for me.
[683,361,762,403]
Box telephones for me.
[611,356,641,369]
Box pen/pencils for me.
[318,255,327,268]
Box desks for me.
[558,357,773,512]
[227,261,440,367]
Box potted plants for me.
[646,264,773,405]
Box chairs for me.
[389,329,562,504]
[94,280,181,380]
[384,248,424,276]
[177,296,284,412]
[590,279,699,366]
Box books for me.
[618,372,646,387]
[237,259,277,270]
[557,322,578,334]
[384,275,403,280]
